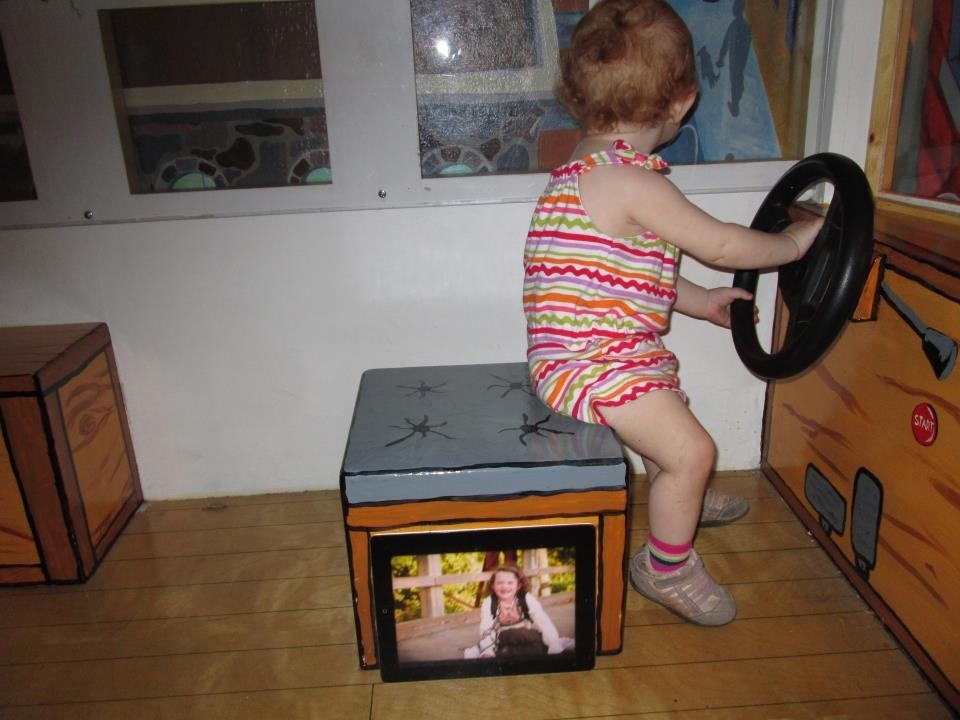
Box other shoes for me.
[698,491,749,526]
[630,544,737,625]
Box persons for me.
[463,567,575,659]
[523,0,824,625]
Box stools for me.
[0,323,142,587]
[341,362,626,669]
